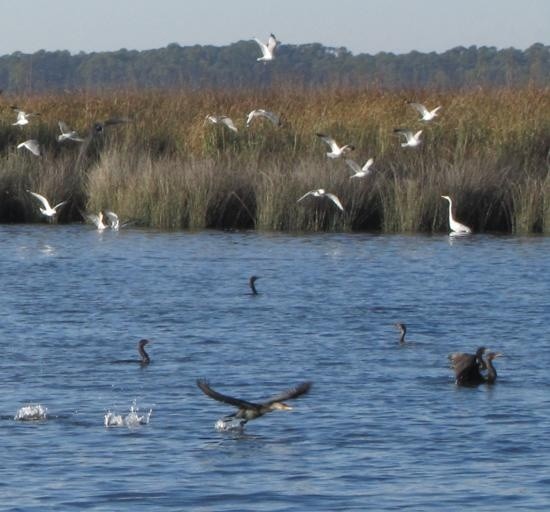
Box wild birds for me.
[246,276,261,295]
[110,339,151,365]
[252,33,282,64]
[11,105,133,230]
[203,101,442,179]
[393,323,407,345]
[194,377,313,428]
[441,195,473,234]
[447,346,501,388]
[296,188,345,212]
